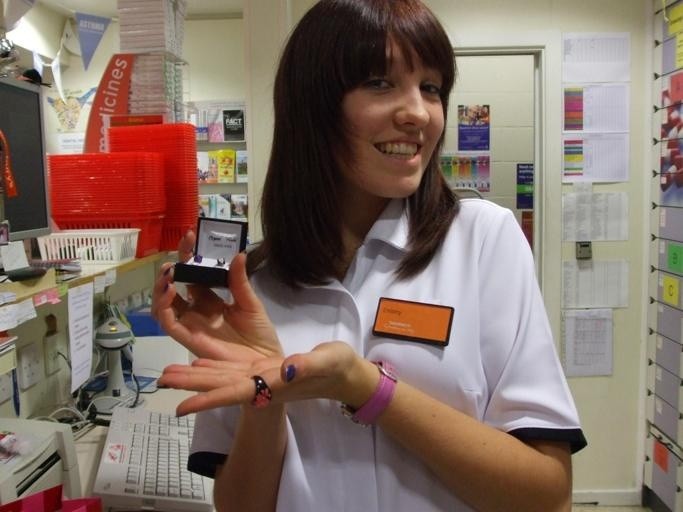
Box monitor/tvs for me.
[0,73,52,280]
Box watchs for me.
[341,358,399,428]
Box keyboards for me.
[92,406,214,512]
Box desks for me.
[0,352,218,511]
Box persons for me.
[149,1,587,512]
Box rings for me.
[249,375,272,410]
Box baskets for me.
[44,122,199,259]
[36,228,142,266]
